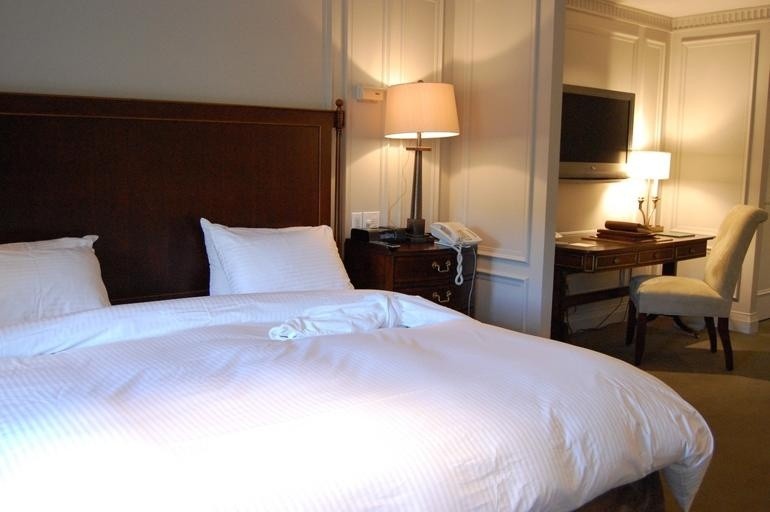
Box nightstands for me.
[343,237,478,318]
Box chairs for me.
[625,203,768,371]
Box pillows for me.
[198,216,356,297]
[0,233,112,331]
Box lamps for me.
[381,82,460,245]
[629,149,671,232]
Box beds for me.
[0,90,714,512]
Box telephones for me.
[429,221,483,286]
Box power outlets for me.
[363,211,380,228]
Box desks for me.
[551,229,716,340]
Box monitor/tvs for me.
[559,83,636,179]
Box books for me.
[581,220,674,246]
[658,230,694,239]
